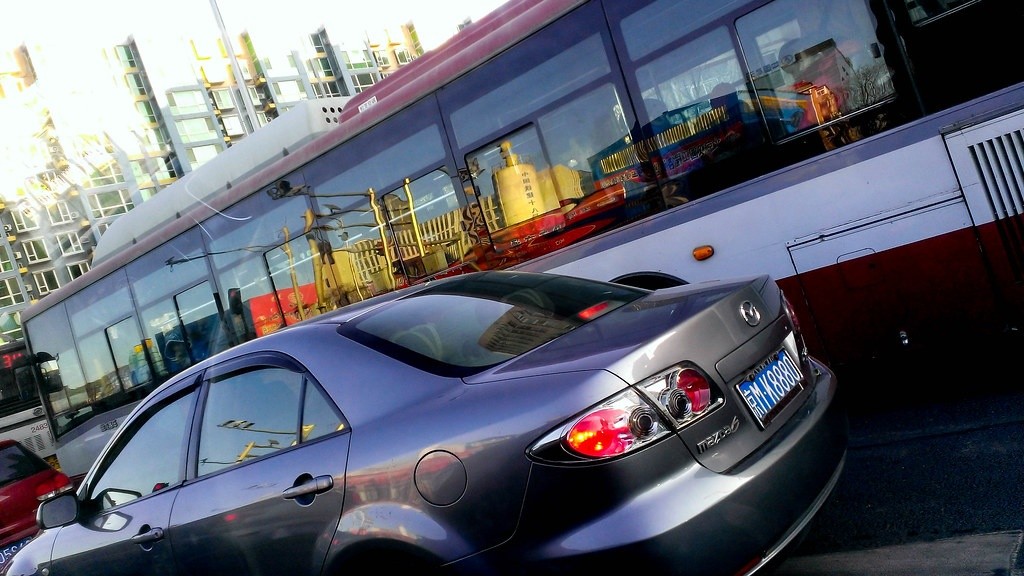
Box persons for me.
[777,34,848,132]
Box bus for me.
[0,342,61,477]
[21,0,1023,480]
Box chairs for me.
[841,51,868,123]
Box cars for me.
[0,269,843,576]
